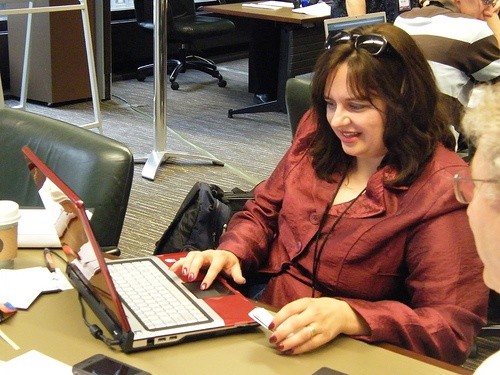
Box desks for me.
[0,249,475,375]
[198,1,331,119]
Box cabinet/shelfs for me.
[6,0,95,106]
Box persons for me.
[328,0,500,375]
[169,22,490,366]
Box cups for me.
[0,200,21,269]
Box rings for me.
[309,326,316,334]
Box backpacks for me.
[153,180,232,256]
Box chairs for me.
[0,107,134,255]
[134,0,235,90]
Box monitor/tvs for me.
[324,11,387,49]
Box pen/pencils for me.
[43,246,56,273]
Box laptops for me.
[21,146,263,353]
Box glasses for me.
[319,31,410,63]
[483,0,496,8]
[451,170,500,205]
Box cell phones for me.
[72,354,152,375]
